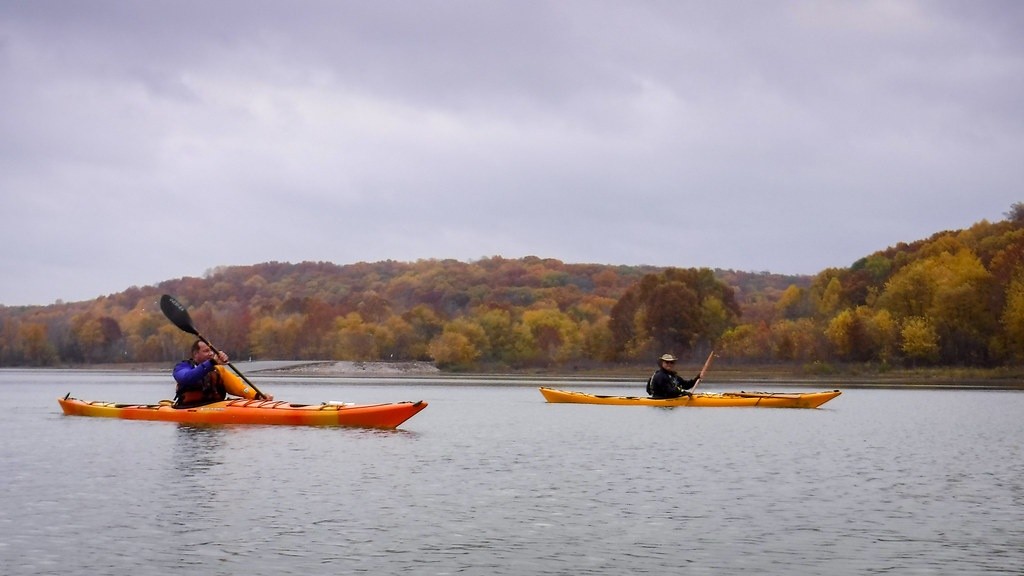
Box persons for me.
[645,354,704,398]
[172,340,274,409]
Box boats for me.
[539,387,841,409]
[59,397,428,429]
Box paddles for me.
[158,293,268,401]
[690,350,716,394]
[726,391,801,399]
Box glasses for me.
[663,360,674,365]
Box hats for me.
[661,354,677,361]
